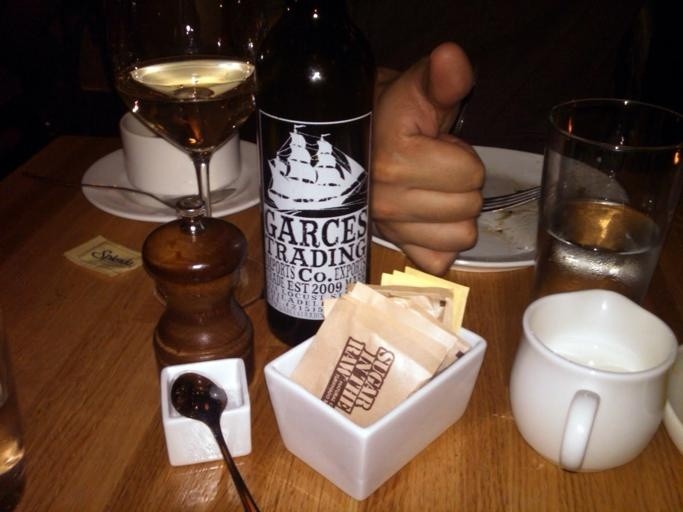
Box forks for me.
[479,186,542,212]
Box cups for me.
[508,287,673,473]
[532,97,680,305]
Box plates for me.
[373,144,558,273]
[76,140,265,224]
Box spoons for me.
[167,374,268,511]
[12,170,237,212]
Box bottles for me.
[260,0,372,348]
[143,195,255,385]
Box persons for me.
[368,42,486,276]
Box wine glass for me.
[113,0,259,216]
